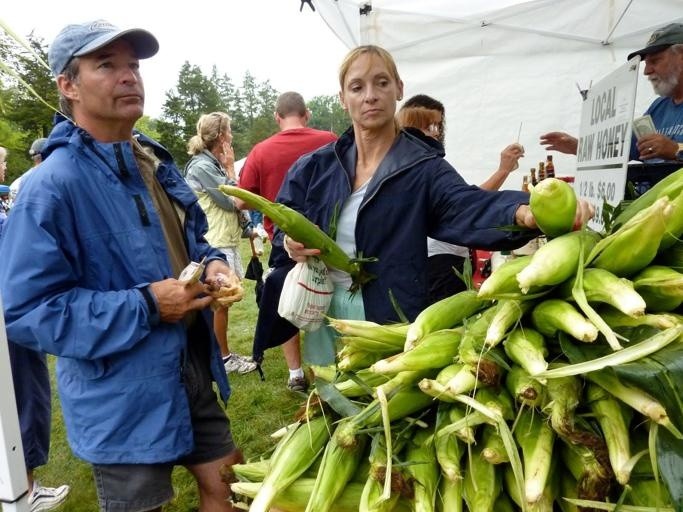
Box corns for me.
[219,165,683,512]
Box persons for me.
[271,44,596,367]
[235,92,339,390]
[243,204,268,258]
[8,135,50,210]
[400,95,445,145]
[0,20,245,512]
[181,112,255,376]
[539,24,682,193]
[391,105,523,305]
[0,146,70,511]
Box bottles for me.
[519,155,557,193]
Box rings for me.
[222,151,229,155]
[647,144,654,154]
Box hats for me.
[49,18,159,78]
[626,23,683,62]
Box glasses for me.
[424,124,438,132]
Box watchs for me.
[674,143,682,162]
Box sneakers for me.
[27,480,71,512]
[286,374,309,393]
[221,353,257,375]
